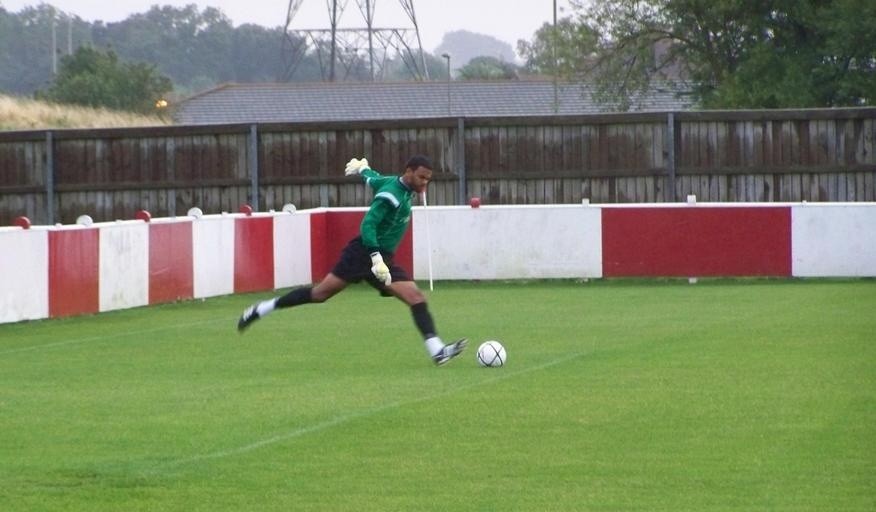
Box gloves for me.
[369,251,392,287]
[345,157,368,177]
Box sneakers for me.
[237,301,261,332]
[431,337,468,367]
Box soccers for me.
[477,341,506,367]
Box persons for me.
[236,154,471,367]
